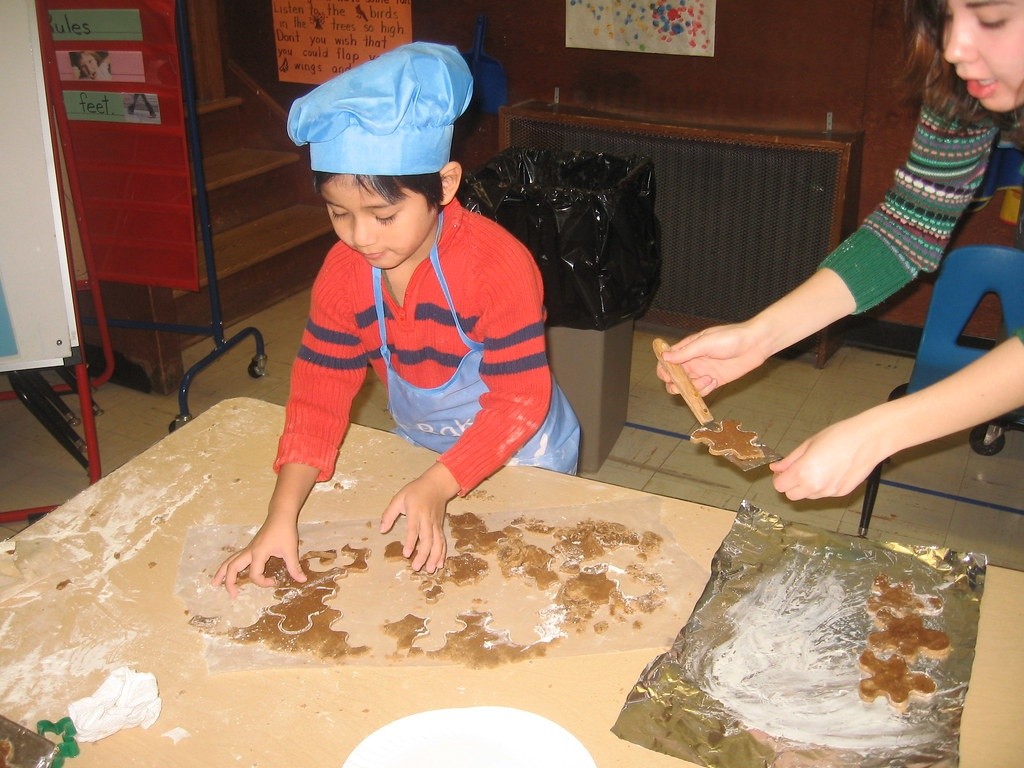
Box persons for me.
[212,43,580,592]
[74,52,111,83]
[654,0,1023,503]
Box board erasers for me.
[458,145,656,474]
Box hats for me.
[287,42,473,176]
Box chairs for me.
[858,243,1024,538]
